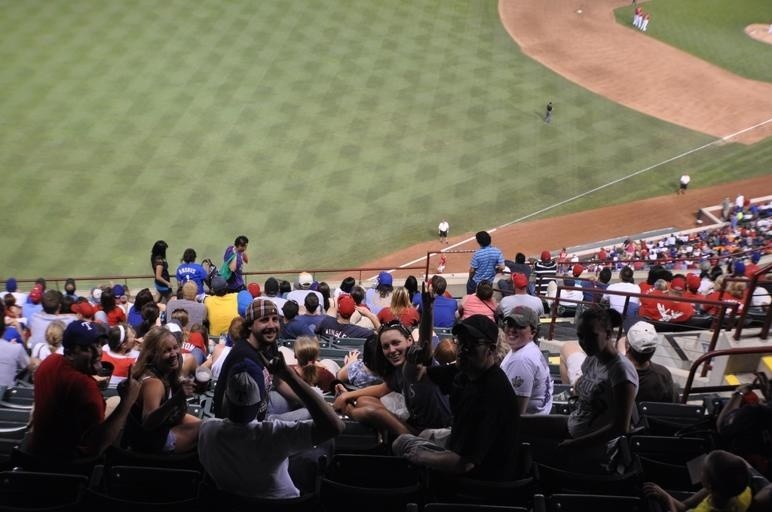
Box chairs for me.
[0,195,772,511]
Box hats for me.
[627,321,657,354]
[298,272,313,287]
[670,273,700,291]
[377,272,392,284]
[92,285,124,299]
[735,262,744,273]
[452,306,539,342]
[512,251,551,288]
[222,358,265,407]
[62,320,105,347]
[8,280,15,292]
[31,284,43,303]
[753,254,759,262]
[245,299,277,320]
[338,294,356,317]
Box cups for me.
[96,361,114,390]
[192,366,213,395]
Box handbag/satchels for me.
[219,253,237,281]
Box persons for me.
[676,171,691,195]
[639,13,650,32]
[631,7,640,27]
[631,1,637,6]
[1,193,771,511]
[636,10,644,29]
[543,101,553,124]
